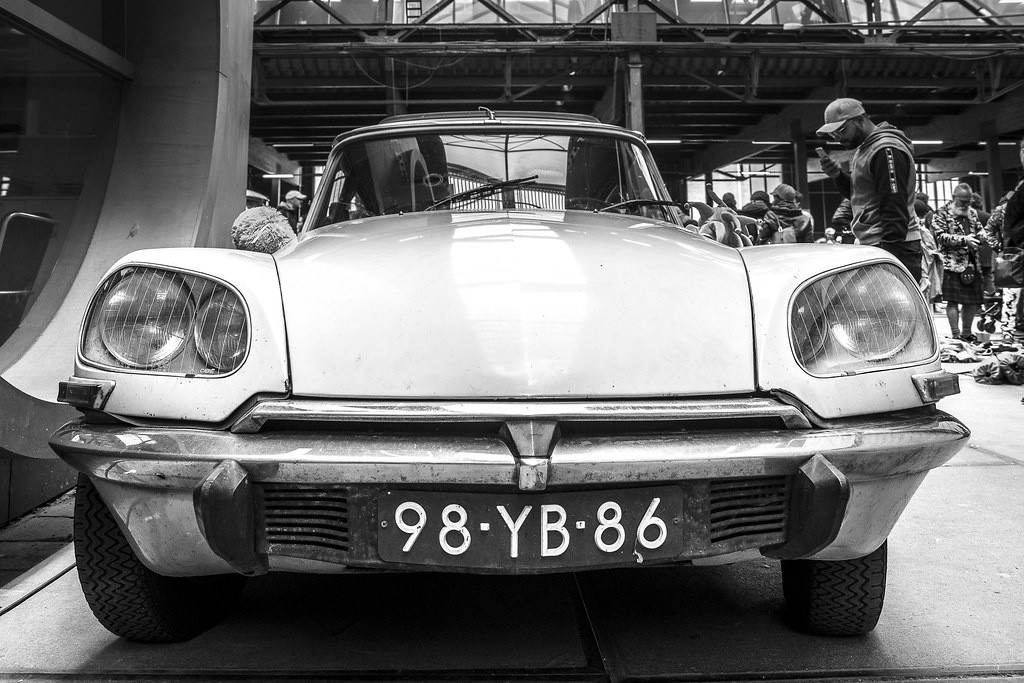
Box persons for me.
[276,135,1023,342]
[816,98,925,284]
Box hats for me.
[769,184,796,202]
[815,97,865,137]
[750,191,769,203]
[285,190,307,200]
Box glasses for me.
[827,119,854,139]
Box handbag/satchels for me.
[994,247,1024,287]
[960,266,975,284]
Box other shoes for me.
[952,335,982,344]
[1002,334,1014,344]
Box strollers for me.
[975,286,1003,334]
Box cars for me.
[45,105,973,645]
